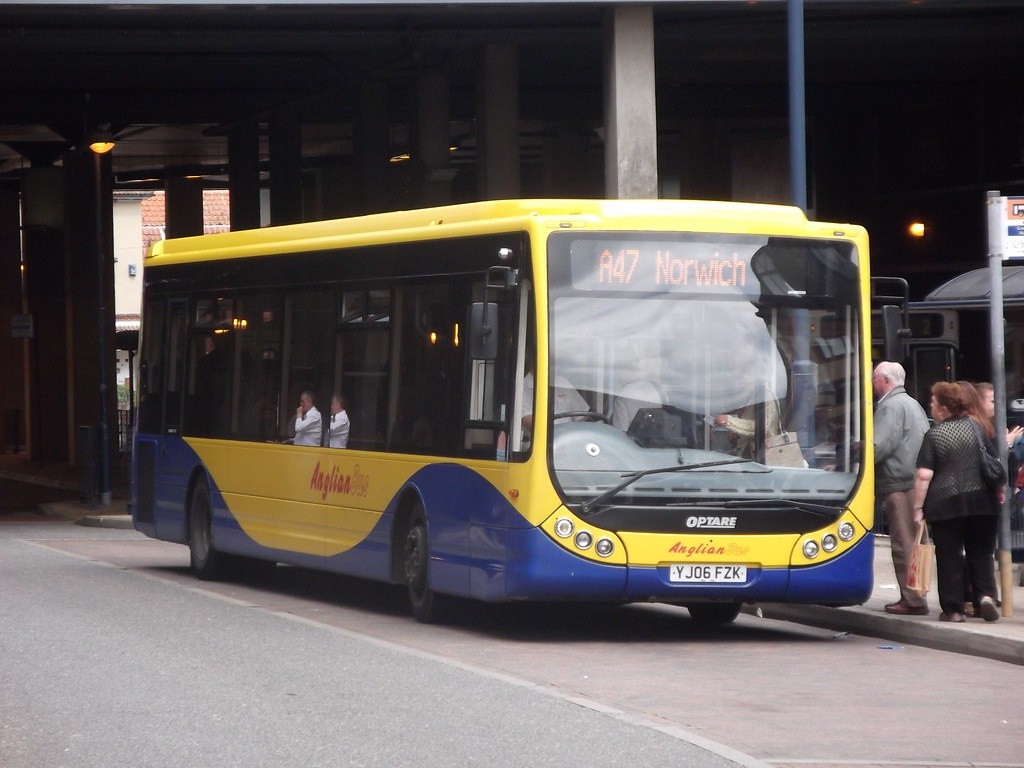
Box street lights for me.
[84,113,117,511]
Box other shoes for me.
[884,597,929,615]
[980,595,1000,622]
[939,611,965,623]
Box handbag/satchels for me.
[766,432,808,471]
[906,521,935,597]
[968,419,1008,489]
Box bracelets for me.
[913,506,922,510]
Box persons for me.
[912,380,1024,624]
[851,361,932,615]
[328,393,350,449]
[614,346,669,434]
[521,342,597,442]
[714,363,781,463]
[285,391,322,449]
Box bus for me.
[129,197,874,626]
[765,306,960,529]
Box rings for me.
[722,421,724,424]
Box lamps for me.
[86,127,117,155]
[384,146,412,165]
